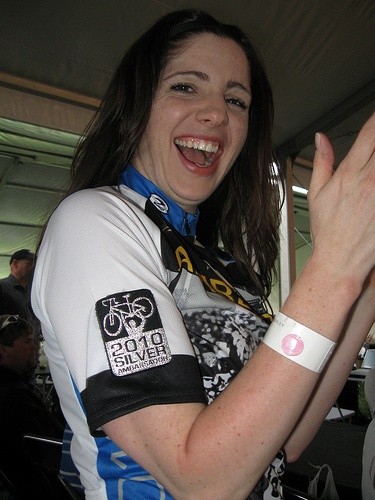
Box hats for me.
[10,249,35,265]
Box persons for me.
[0,248,75,500]
[31,13,375,500]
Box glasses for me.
[1,314,21,328]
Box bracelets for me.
[258,312,334,373]
